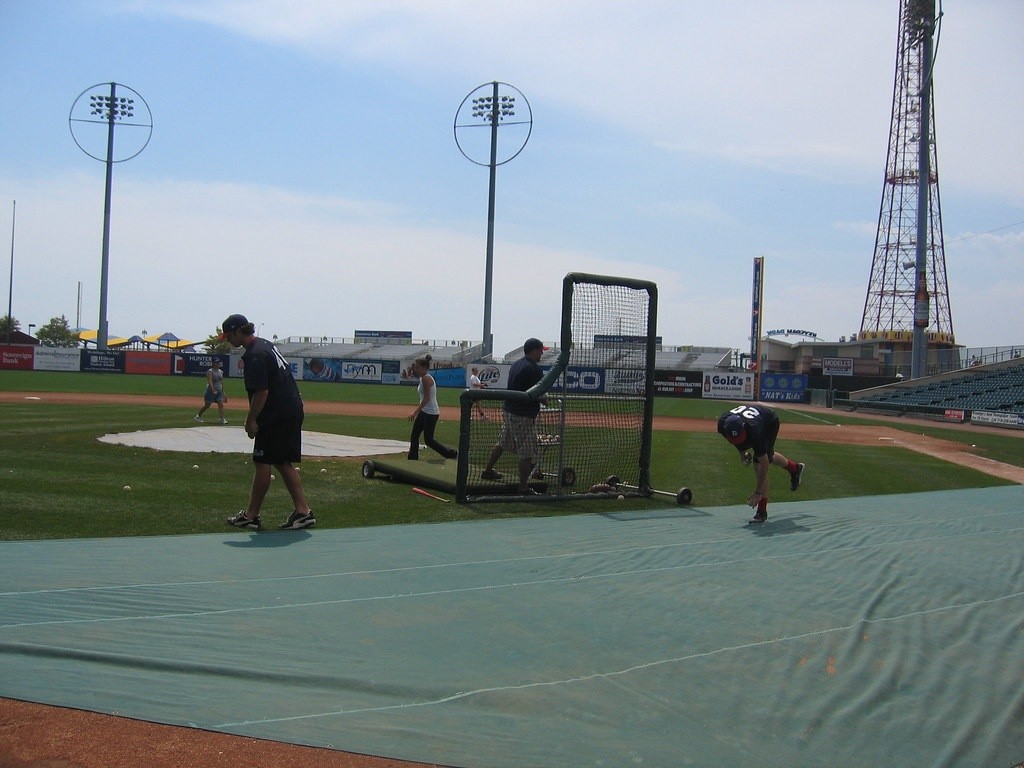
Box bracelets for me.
[754,489,761,494]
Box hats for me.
[524,338,549,353]
[212,356,220,364]
[217,314,248,341]
[724,414,748,444]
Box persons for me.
[481,338,550,496]
[717,403,805,524]
[470,368,489,421]
[194,357,228,424]
[217,314,317,530]
[408,354,457,460]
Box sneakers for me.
[195,414,203,422]
[749,510,768,524]
[227,510,262,528]
[219,417,228,424]
[480,467,504,480]
[518,485,542,497]
[791,463,805,491]
[278,509,316,529]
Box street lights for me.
[90,93,135,351]
[141,330,148,337]
[28,324,36,336]
[472,95,517,361]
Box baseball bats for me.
[411,487,451,503]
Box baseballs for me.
[294,466,301,473]
[536,433,561,443]
[270,474,276,481]
[319,468,328,475]
[743,452,752,466]
[617,495,625,502]
[214,390,217,394]
[746,498,757,507]
[192,464,199,471]
[123,485,132,492]
